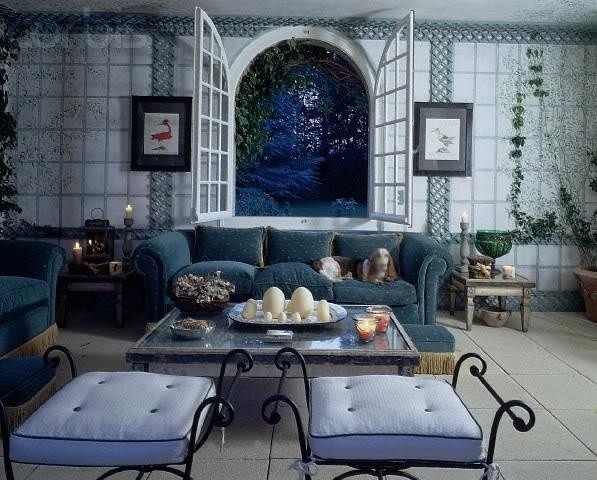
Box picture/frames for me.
[130,94,193,172]
[413,102,474,177]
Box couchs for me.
[133,225,453,324]
[0,237,67,359]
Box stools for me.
[399,324,456,376]
[1,347,254,480]
[259,347,537,479]
[1,336,77,433]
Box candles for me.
[73,241,83,264]
[462,212,468,223]
[125,204,133,218]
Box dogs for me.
[310,247,399,285]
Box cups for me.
[352,314,378,343]
[365,306,392,333]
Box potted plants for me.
[560,155,597,323]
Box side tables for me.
[57,265,137,329]
[449,268,539,333]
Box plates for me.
[169,319,216,339]
[228,299,347,328]
